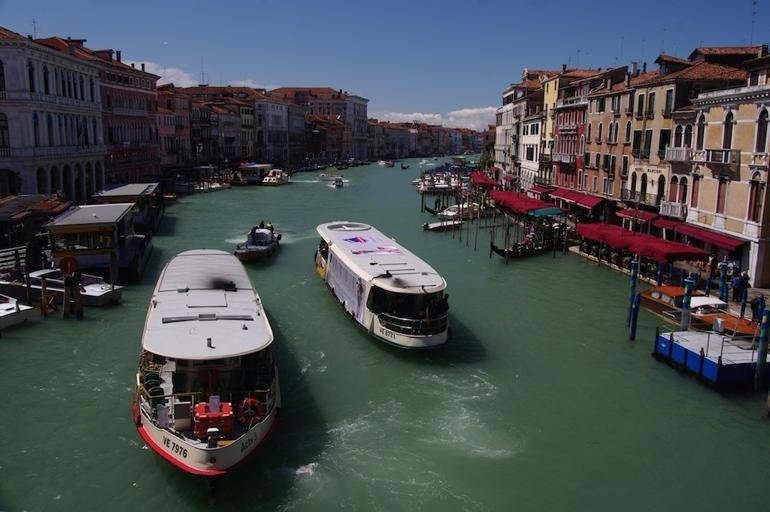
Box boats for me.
[0,292,33,330]
[134,250,281,482]
[334,157,410,170]
[262,169,290,187]
[231,229,282,262]
[319,173,346,181]
[423,201,484,221]
[640,285,762,339]
[423,217,464,231]
[333,177,344,188]
[22,267,123,308]
[315,220,450,353]
[491,234,562,258]
[413,158,462,193]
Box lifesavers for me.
[237,398,265,426]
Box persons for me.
[419,157,767,322]
[266,220,273,234]
[313,221,450,337]
[259,220,264,230]
